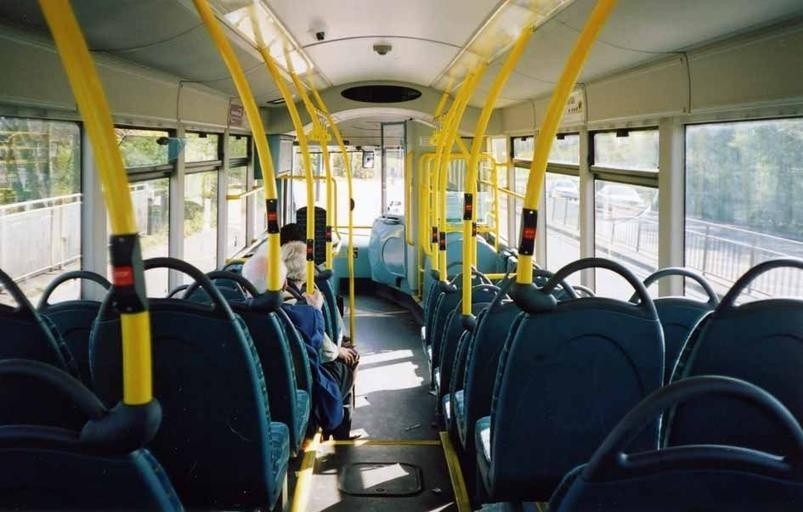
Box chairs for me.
[421,257,803,512]
[0,257,339,512]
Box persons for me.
[242,254,353,448]
[281,241,360,414]
[280,222,347,338]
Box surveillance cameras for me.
[373,42,393,55]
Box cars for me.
[549,180,579,200]
[596,185,639,212]
